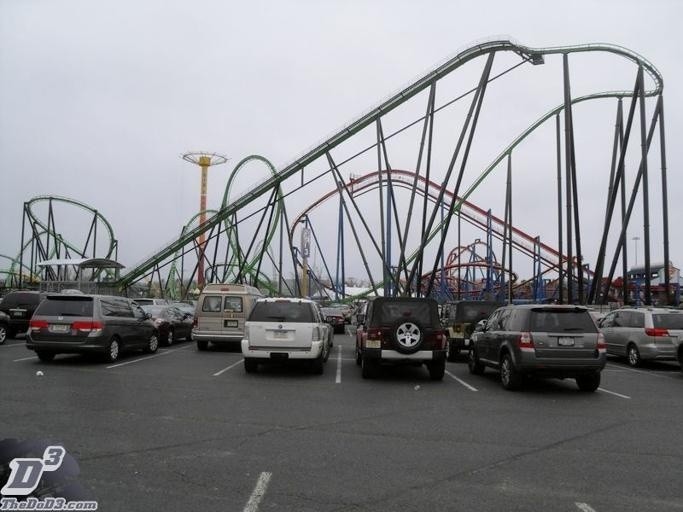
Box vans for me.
[190,281,264,351]
[23,292,160,362]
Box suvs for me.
[354,295,448,382]
[595,306,682,367]
[241,296,334,378]
[439,300,510,363]
[467,301,607,393]
[0,288,59,340]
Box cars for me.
[0,310,11,344]
[319,307,345,335]
[333,304,352,325]
[133,297,195,346]
